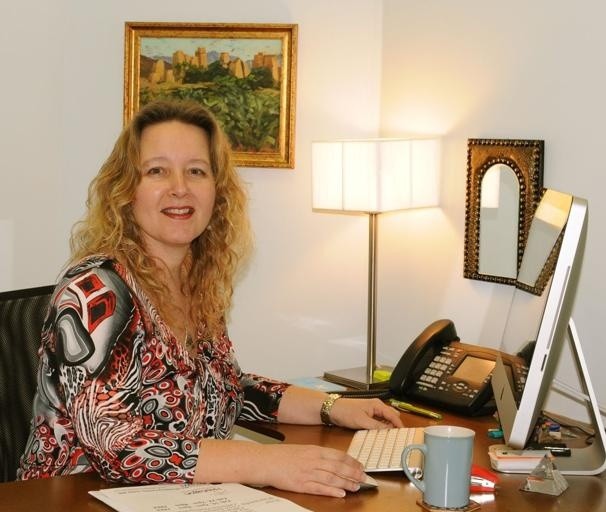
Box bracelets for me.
[320,391,343,428]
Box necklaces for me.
[169,294,195,353]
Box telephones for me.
[387,318,529,417]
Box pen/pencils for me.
[388,397,442,420]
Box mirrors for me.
[462,138,545,287]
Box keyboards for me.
[342,427,424,479]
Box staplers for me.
[468,464,502,493]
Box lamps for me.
[310,135,444,394]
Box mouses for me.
[354,473,378,491]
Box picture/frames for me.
[122,20,299,171]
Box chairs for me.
[0,285,57,484]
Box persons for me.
[13,103,404,499]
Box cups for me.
[400,425,476,509]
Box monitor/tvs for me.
[490,191,606,476]
